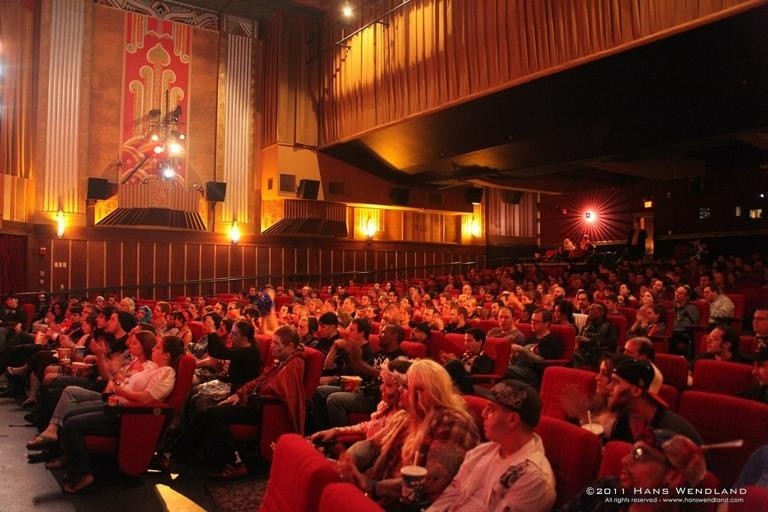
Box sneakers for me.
[5,365,25,380]
[22,397,39,408]
[27,451,49,462]
[45,459,68,469]
[24,411,46,423]
[212,461,248,481]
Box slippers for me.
[61,476,96,495]
[26,433,62,451]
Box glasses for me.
[625,443,678,473]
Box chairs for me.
[14,252,768,510]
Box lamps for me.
[147,103,187,183]
[365,214,375,242]
[57,208,64,237]
[232,218,240,245]
[342,6,390,30]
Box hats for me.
[633,427,707,482]
[113,310,137,333]
[408,320,432,338]
[472,378,542,429]
[611,356,669,411]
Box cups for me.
[401,466,427,502]
[341,375,363,394]
[57,345,84,364]
[581,422,604,443]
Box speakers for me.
[393,188,410,205]
[88,178,108,199]
[207,182,226,201]
[468,187,483,203]
[299,179,320,199]
[510,191,522,203]
